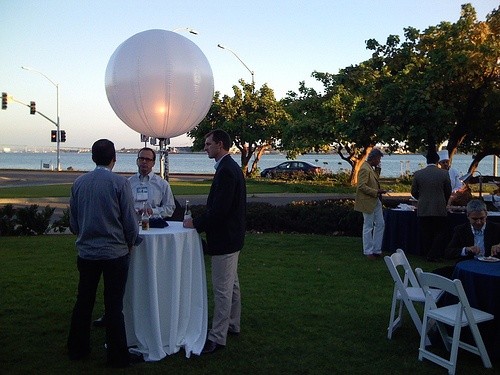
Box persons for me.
[67,138,140,362]
[445,199,500,261]
[354,149,388,261]
[437,149,463,193]
[411,152,452,262]
[93,148,176,326]
[183,130,247,352]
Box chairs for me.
[384,249,443,346]
[415,267,494,375]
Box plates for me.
[478,257,499,261]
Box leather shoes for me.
[201,341,224,353]
[94,317,105,325]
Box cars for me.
[260,160,322,181]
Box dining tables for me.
[427,258,500,367]
[381,206,500,258]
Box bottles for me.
[184,201,191,220]
[141,204,149,231]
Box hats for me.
[438,150,449,162]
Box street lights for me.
[174,28,199,36]
[216,44,254,93]
[20,65,61,171]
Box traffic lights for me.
[2,92,8,110]
[61,130,66,142]
[51,130,57,142]
[140,134,150,142]
[30,101,36,114]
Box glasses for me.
[138,157,152,161]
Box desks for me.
[122,221,208,361]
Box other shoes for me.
[367,254,384,259]
